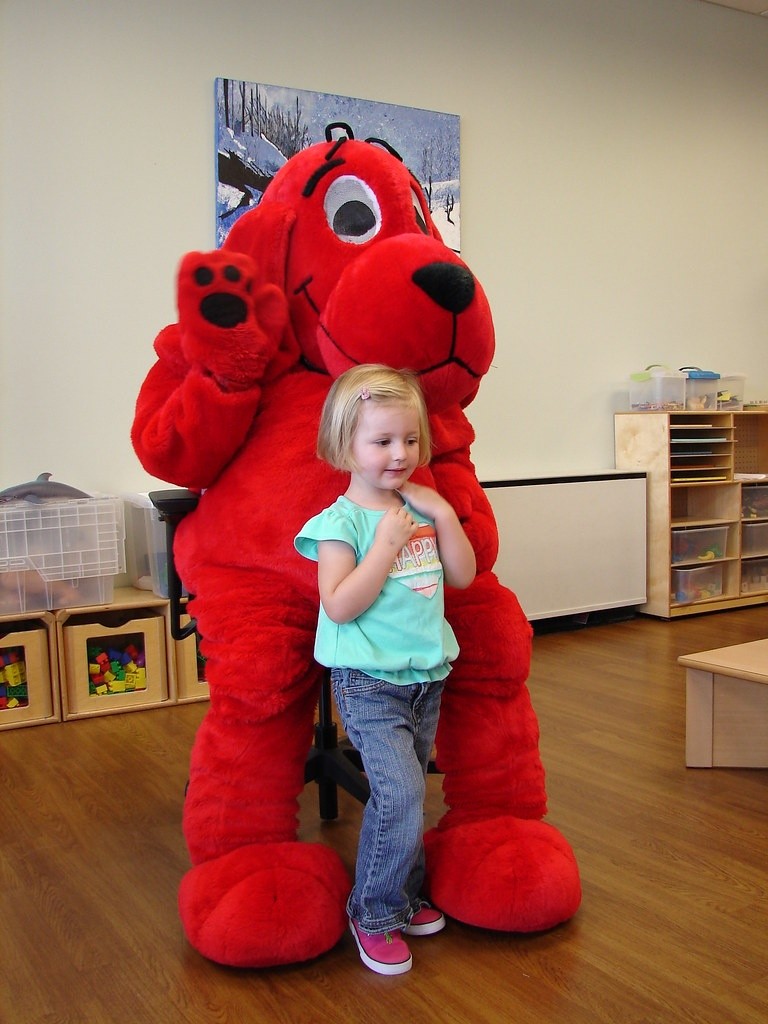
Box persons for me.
[293,362,477,977]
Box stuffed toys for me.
[128,120,584,971]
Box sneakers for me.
[401,907,446,935]
[349,916,412,975]
